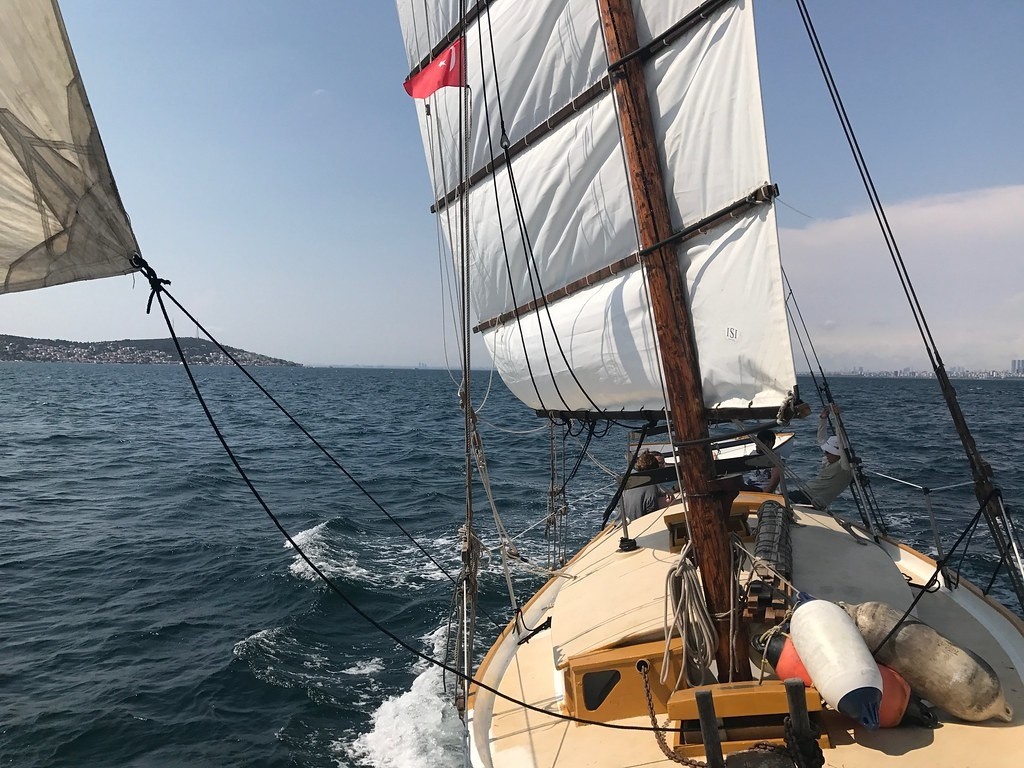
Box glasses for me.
[658,460,665,464]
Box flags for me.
[403,36,464,99]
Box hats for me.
[821,436,840,455]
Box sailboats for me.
[1,0,1024,768]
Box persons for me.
[623,451,665,521]
[741,430,780,493]
[786,409,851,511]
